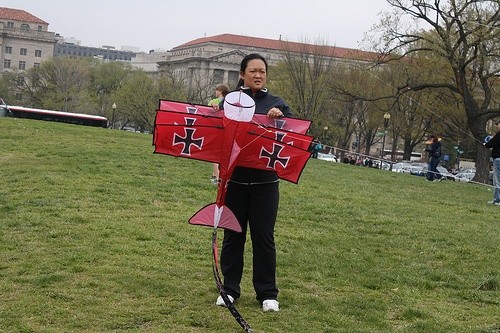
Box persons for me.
[209,53,293,313]
[307,136,374,168]
[207,84,230,183]
[482,119,500,206]
[423,134,444,184]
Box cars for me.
[316,151,477,183]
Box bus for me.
[0,104,108,128]
[379,149,422,164]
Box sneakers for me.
[263,299,279,312]
[216,294,235,306]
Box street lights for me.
[380,112,391,169]
[111,103,116,129]
[454,143,464,170]
[323,126,329,145]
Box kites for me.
[153,90,314,331]
